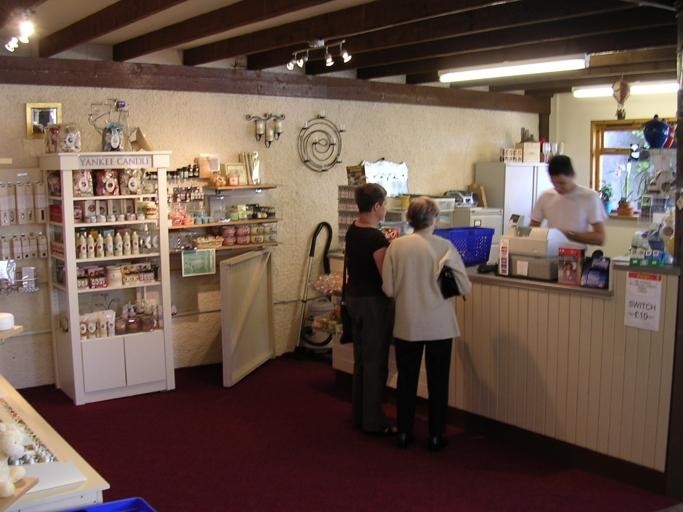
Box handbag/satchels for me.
[440,267,461,298]
[340,302,354,344]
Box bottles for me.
[81,310,116,338]
[80,231,139,259]
[643,114,675,149]
[174,185,201,203]
[178,165,199,179]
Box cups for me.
[210,194,225,221]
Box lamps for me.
[284,37,353,69]
[4,7,39,52]
[243,113,287,147]
[299,110,346,173]
[570,77,683,98]
[436,52,592,82]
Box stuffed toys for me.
[0,423,26,498]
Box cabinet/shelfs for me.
[471,159,556,241]
[168,182,279,254]
[41,150,177,405]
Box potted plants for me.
[617,190,636,217]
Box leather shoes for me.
[369,425,440,452]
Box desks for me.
[0,375,111,512]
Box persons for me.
[344,183,399,434]
[382,196,471,449]
[529,155,608,245]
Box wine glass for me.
[542,142,557,163]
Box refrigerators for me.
[475,162,554,233]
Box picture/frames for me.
[25,102,62,140]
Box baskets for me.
[433,227,495,264]
